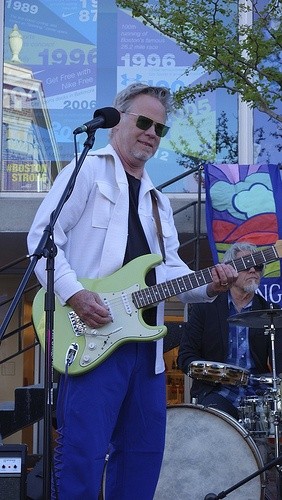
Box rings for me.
[220,282,228,286]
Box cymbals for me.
[190,363,246,384]
[226,309,282,328]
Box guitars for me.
[29,239,282,376]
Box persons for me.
[27,85,237,500]
[177,241,282,421]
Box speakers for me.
[0,443,27,499]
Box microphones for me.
[73,106,121,134]
[207,495,217,500]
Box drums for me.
[238,395,282,439]
[101,403,267,500]
[188,360,252,387]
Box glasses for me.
[121,111,170,138]
[244,264,265,273]
